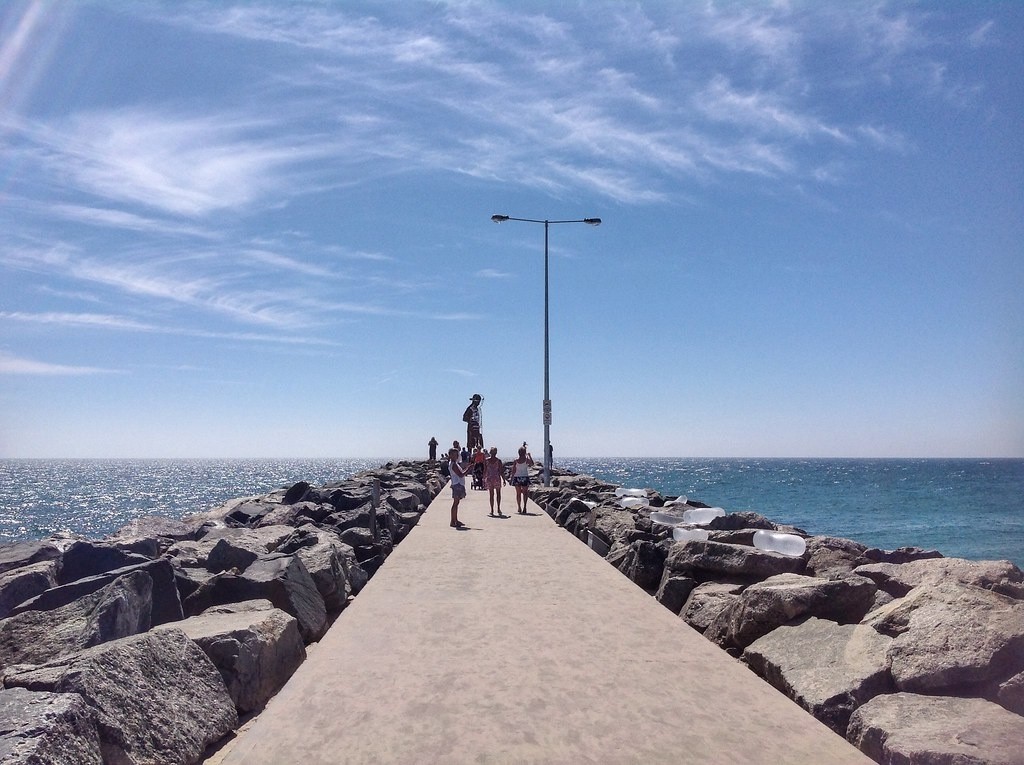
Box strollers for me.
[471,462,484,490]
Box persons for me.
[448,449,473,527]
[521,441,527,447]
[453,441,461,451]
[462,394,483,452]
[473,446,489,476]
[440,453,448,460]
[549,440,553,470]
[509,447,534,514]
[428,437,438,460]
[461,447,470,476]
[483,447,506,516]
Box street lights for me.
[491,214,602,487]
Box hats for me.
[470,393,482,402]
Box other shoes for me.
[518,508,521,513]
[498,510,502,515]
[450,521,465,527]
[523,508,527,513]
[489,512,494,517]
